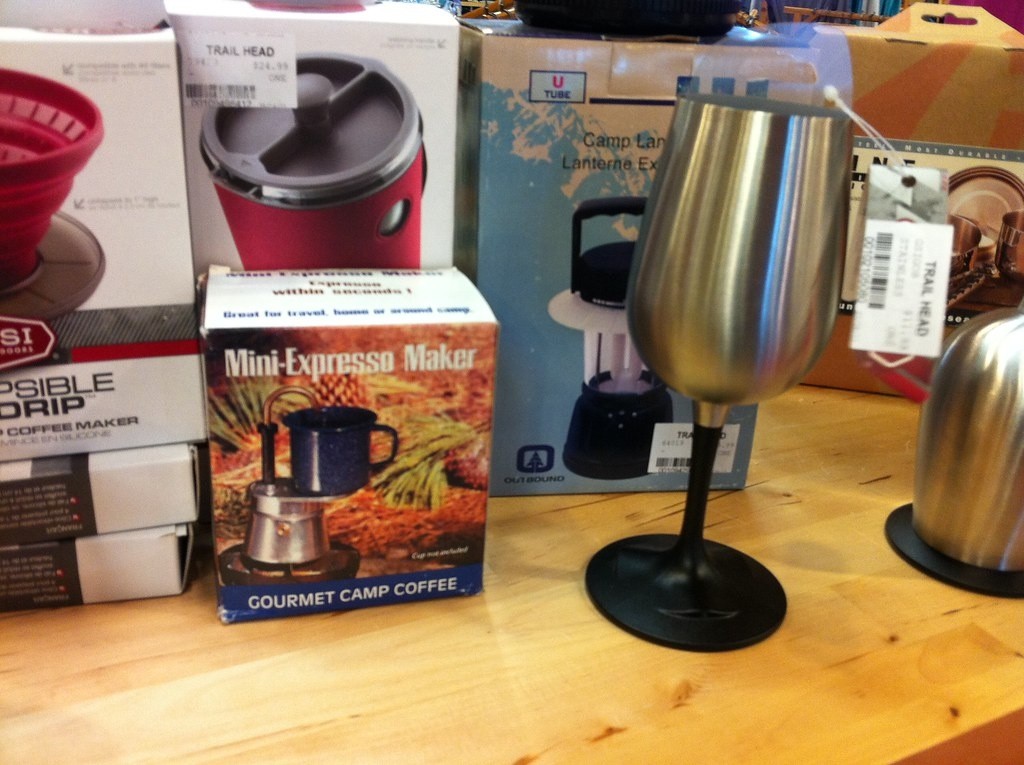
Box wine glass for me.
[584,90,852,653]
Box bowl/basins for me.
[0,68,105,285]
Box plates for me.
[0,206,106,331]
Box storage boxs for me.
[0,340,207,465]
[0,0,205,350]
[0,524,187,612]
[0,442,199,548]
[798,0,1024,397]
[463,16,817,498]
[197,266,498,628]
[165,0,464,267]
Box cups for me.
[195,51,429,270]
[278,403,402,497]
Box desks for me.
[0,384,1024,764]
